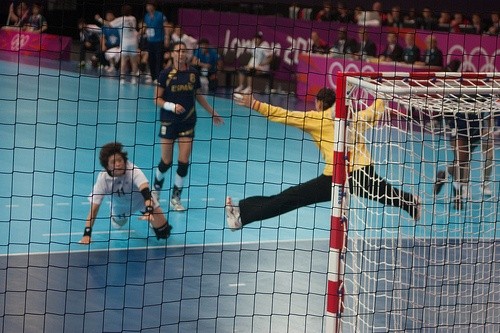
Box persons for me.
[436,94,500,209]
[226,87,422,231]
[154,41,223,210]
[77,143,172,244]
[380,33,401,61]
[235,35,273,94]
[312,32,329,54]
[444,59,460,72]
[422,36,443,66]
[402,32,420,61]
[331,27,357,52]
[78,0,171,83]
[10,0,48,33]
[289,0,500,36]
[164,26,197,69]
[192,39,217,94]
[358,28,376,56]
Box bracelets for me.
[84,227,92,237]
[146,206,153,212]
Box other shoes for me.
[152,188,160,200]
[234,86,252,93]
[452,185,464,210]
[414,194,422,222]
[78,60,160,86]
[225,196,242,232]
[479,185,492,197]
[170,197,186,212]
[462,186,472,198]
[434,170,445,194]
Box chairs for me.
[86,40,278,93]
